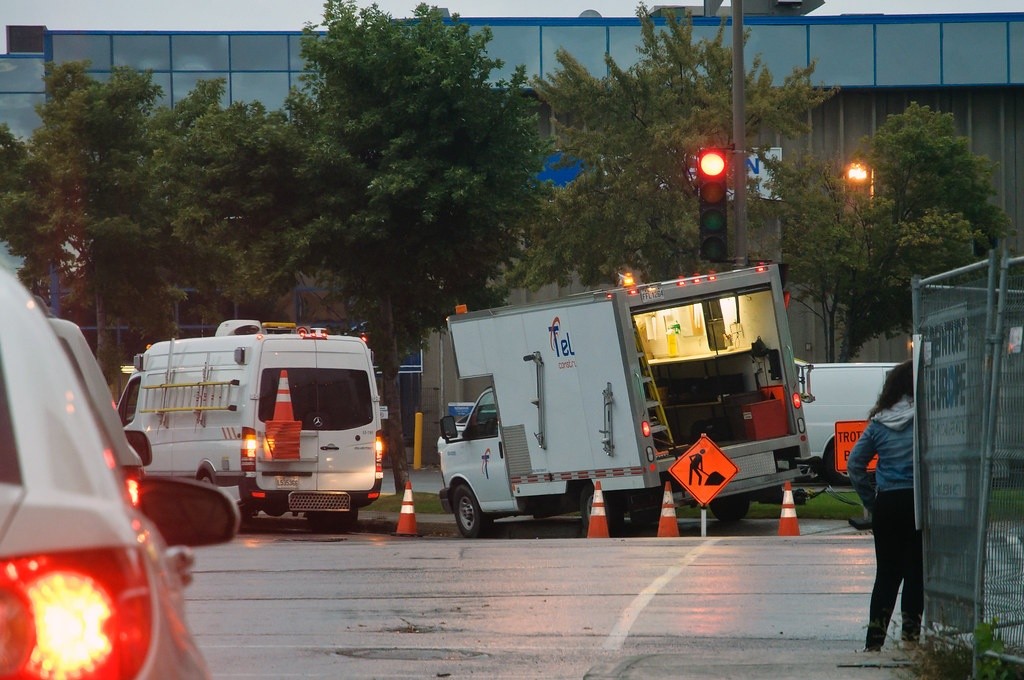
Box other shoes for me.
[863,646,882,654]
[899,640,919,650]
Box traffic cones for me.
[656,481,681,538]
[388,480,418,539]
[586,480,611,538]
[777,480,801,536]
[259,368,303,459]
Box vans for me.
[117,319,386,531]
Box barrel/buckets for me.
[724,391,761,441]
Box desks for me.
[649,401,727,439]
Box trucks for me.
[435,263,832,541]
[789,363,910,486]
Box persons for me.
[847,358,924,652]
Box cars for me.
[0,265,240,680]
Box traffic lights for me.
[695,148,730,264]
